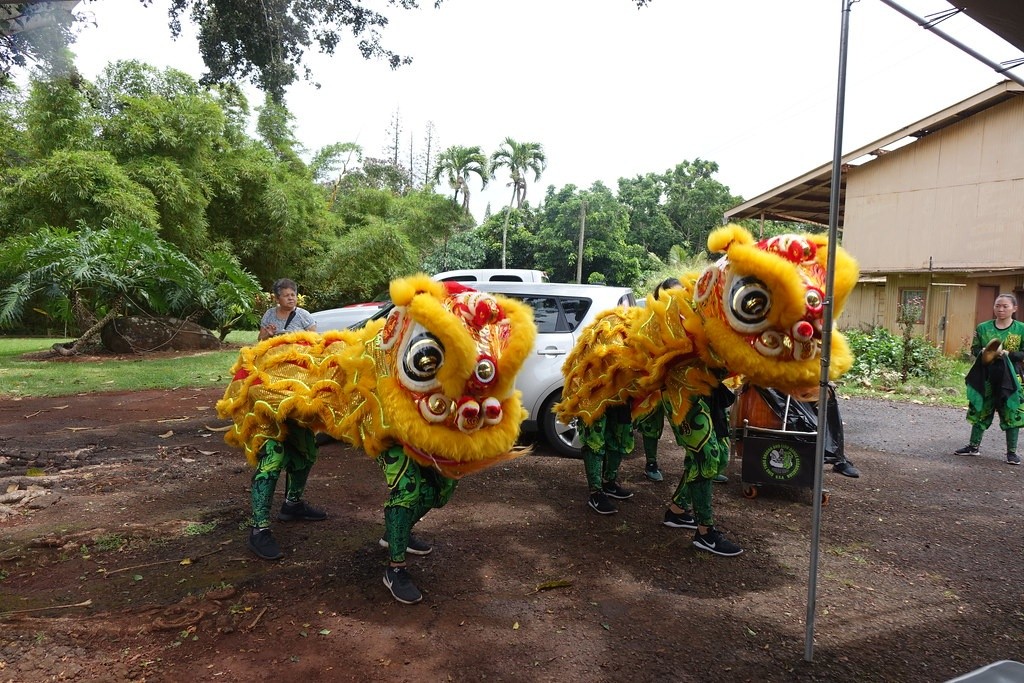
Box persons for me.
[375,444,459,603]
[248,418,328,559]
[660,384,744,556]
[258,279,317,342]
[575,403,634,513]
[954,294,1024,464]
[635,403,665,482]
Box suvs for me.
[307,268,652,460]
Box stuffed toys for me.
[214,275,537,480]
[550,224,859,427]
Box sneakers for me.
[663,509,698,529]
[383,566,422,604]
[643,464,664,482]
[601,479,633,499]
[693,526,743,557]
[954,444,980,456]
[588,491,618,514]
[246,528,284,560]
[712,474,728,483]
[280,499,326,521]
[1006,452,1021,465]
[379,530,432,555]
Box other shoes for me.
[833,461,859,478]
